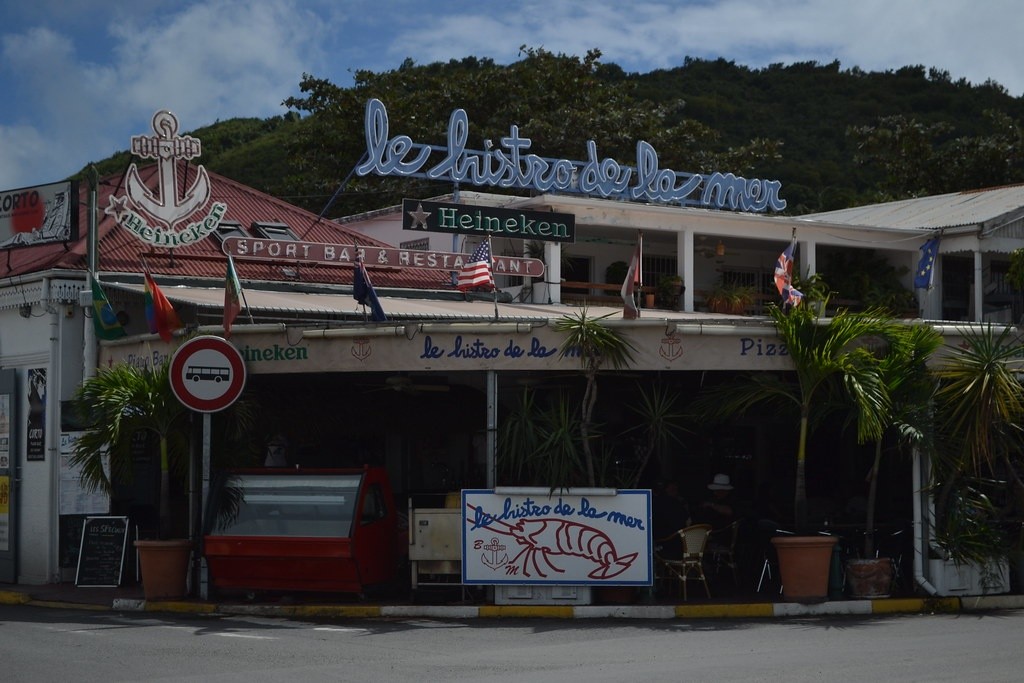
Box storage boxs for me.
[928,555,1011,596]
[495,584,593,606]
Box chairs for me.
[646,523,713,604]
[693,514,750,598]
[756,516,789,597]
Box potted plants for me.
[707,269,759,314]
[62,331,270,602]
[493,323,701,605]
[660,275,683,295]
[689,294,908,600]
[795,320,953,598]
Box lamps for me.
[675,324,785,337]
[926,322,1018,336]
[280,267,297,280]
[417,322,531,334]
[302,326,408,339]
[99,328,188,347]
[546,318,669,327]
[197,322,288,334]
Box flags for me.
[222,256,242,340]
[144,267,184,343]
[774,240,804,307]
[91,277,128,340]
[621,243,640,318]
[353,252,387,322]
[914,238,941,290]
[458,238,494,292]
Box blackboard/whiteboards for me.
[75,516,139,588]
[461,488,653,586]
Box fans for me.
[703,239,741,259]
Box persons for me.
[652,470,744,599]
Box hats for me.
[707,474,734,489]
